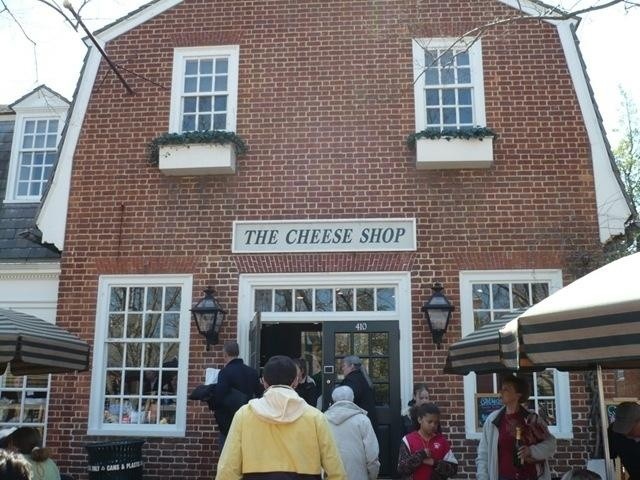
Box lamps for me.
[189,288,228,351]
[420,280,456,349]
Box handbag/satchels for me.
[512,412,548,466]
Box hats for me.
[612,402,640,434]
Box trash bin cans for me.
[86,439,146,480]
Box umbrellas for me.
[0,306,90,376]
[442,304,535,374]
[498,247,640,372]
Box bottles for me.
[512,425,526,468]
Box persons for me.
[142,369,162,404]
[189,340,265,452]
[0,375,29,423]
[328,355,376,433]
[258,367,271,390]
[396,403,459,480]
[475,376,557,480]
[294,358,318,408]
[560,467,603,480]
[161,372,177,405]
[0,426,62,480]
[606,401,640,480]
[321,385,382,480]
[399,384,444,436]
[214,355,348,480]
